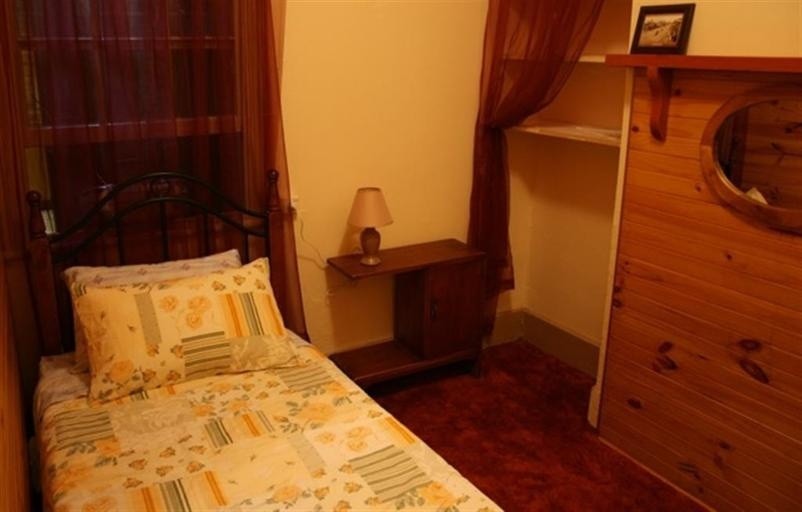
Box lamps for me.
[347,187,393,266]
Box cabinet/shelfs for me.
[495,53,625,149]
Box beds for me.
[21,169,507,512]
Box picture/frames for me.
[630,3,696,56]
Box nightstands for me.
[329,239,488,385]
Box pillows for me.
[61,248,243,374]
[68,256,305,410]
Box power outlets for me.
[282,195,301,223]
